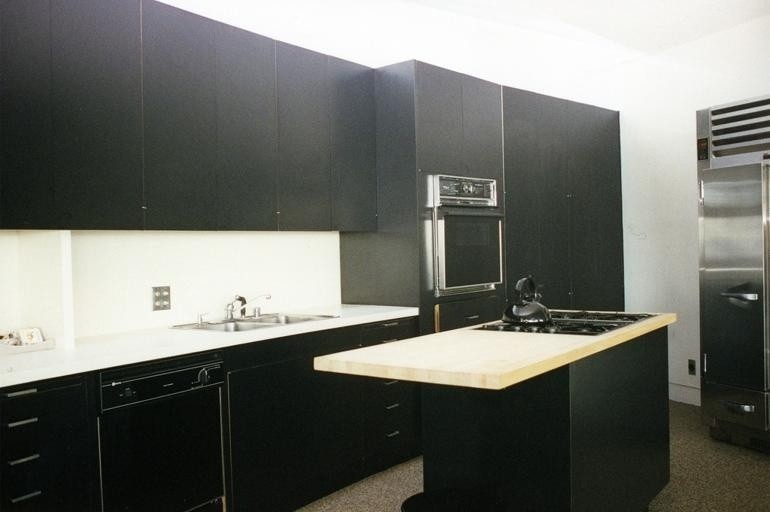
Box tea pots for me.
[503,275,552,330]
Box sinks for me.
[252,314,342,323]
[171,322,271,333]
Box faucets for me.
[226,294,271,319]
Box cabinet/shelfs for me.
[141,0,277,232]
[501,86,624,314]
[278,41,377,231]
[225,357,370,512]
[1,0,142,229]
[414,62,503,182]
[0,388,103,512]
[360,318,424,468]
[428,297,503,333]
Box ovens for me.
[698,98,769,451]
[92,348,233,511]
[429,175,503,295]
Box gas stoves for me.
[475,310,654,337]
